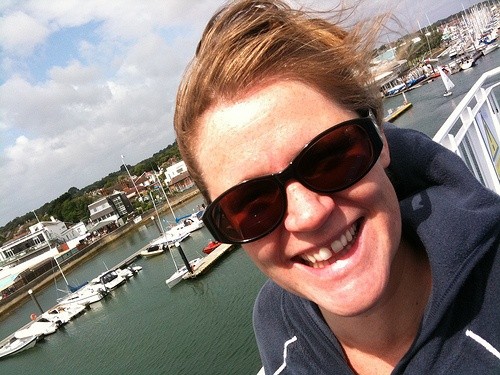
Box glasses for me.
[197,107,383,244]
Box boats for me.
[39,303,86,324]
[148,191,202,290]
[15,322,57,338]
[165,212,207,233]
[147,233,191,246]
[59,284,110,304]
[141,245,166,259]
[0,335,38,358]
[88,266,143,289]
[202,238,222,255]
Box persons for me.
[173,0,500,375]
